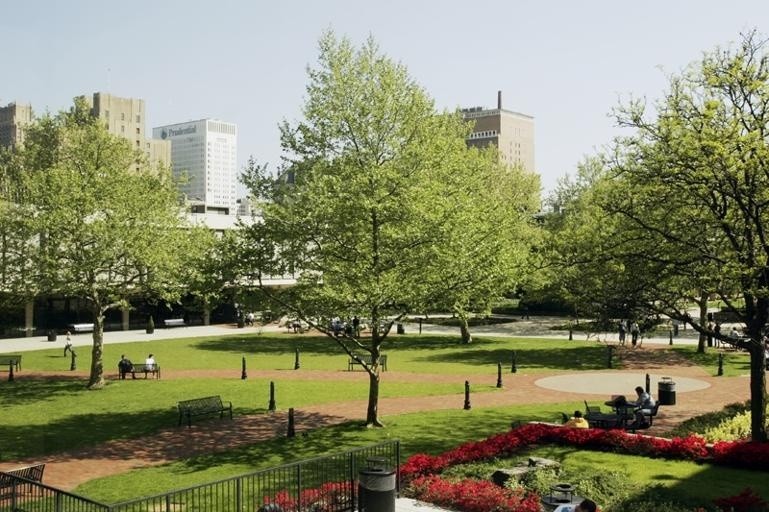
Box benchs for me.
[0,354,22,371]
[0,463,45,501]
[177,395,233,429]
[118,363,160,380]
[348,353,388,372]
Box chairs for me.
[561,393,662,438]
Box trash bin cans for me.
[541,482,585,512]
[48,328,56,341]
[359,456,396,512]
[658,377,676,405]
[397,324,404,334]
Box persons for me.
[730,326,738,348]
[331,316,361,338]
[617,318,640,348]
[707,324,712,347]
[714,322,721,348]
[63,331,72,357]
[118,355,138,379]
[671,313,679,337]
[566,410,589,429]
[632,386,656,428]
[143,354,157,379]
[246,312,254,324]
[552,499,596,512]
